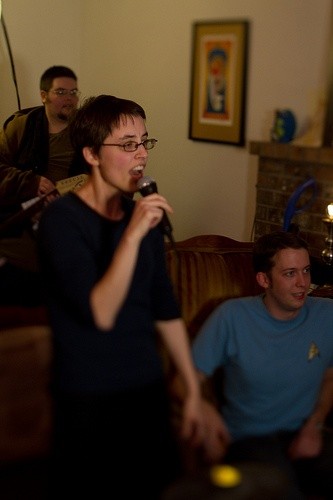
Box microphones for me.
[137,175,177,247]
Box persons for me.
[171,230,333,500]
[0,66,91,275]
[36,95,206,500]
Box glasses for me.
[101,139,157,152]
[48,89,77,96]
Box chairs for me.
[165,235,257,409]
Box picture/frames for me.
[186,17,249,146]
